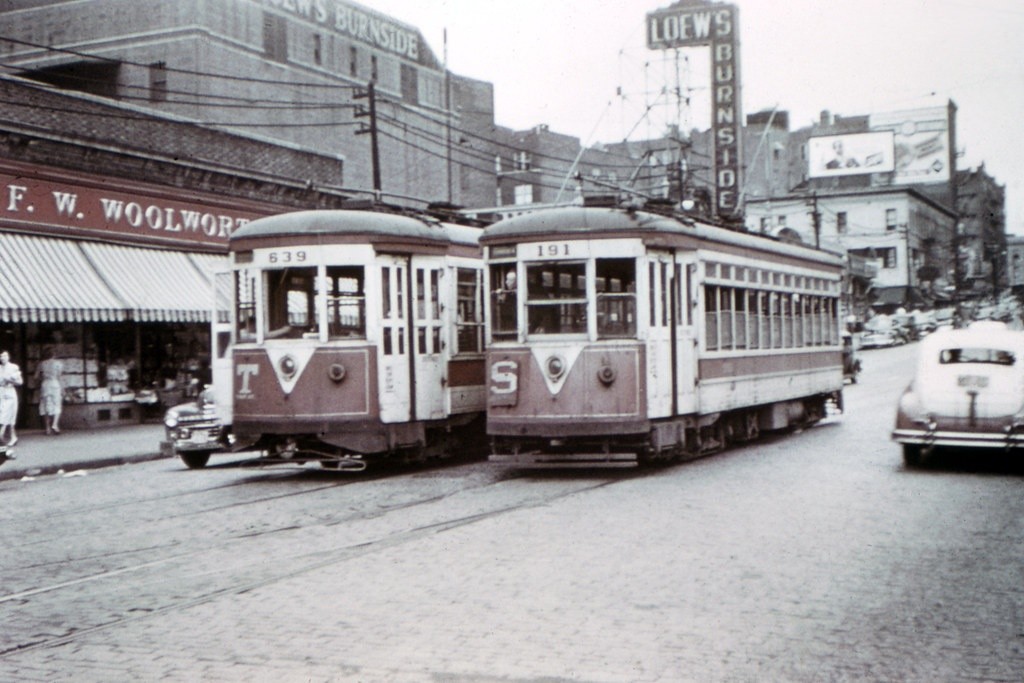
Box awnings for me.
[0,234,462,331]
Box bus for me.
[220,210,488,476]
[479,204,849,467]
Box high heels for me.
[6,437,19,447]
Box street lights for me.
[991,251,1007,299]
[442,29,472,204]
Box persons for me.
[496,271,551,334]
[34,346,63,434]
[825,140,860,168]
[0,350,23,446]
[897,300,921,339]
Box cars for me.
[935,307,964,330]
[860,314,910,349]
[842,331,861,384]
[161,384,300,471]
[960,296,1020,323]
[913,310,939,336]
[891,319,1024,471]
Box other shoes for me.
[52,427,61,435]
[46,426,52,435]
